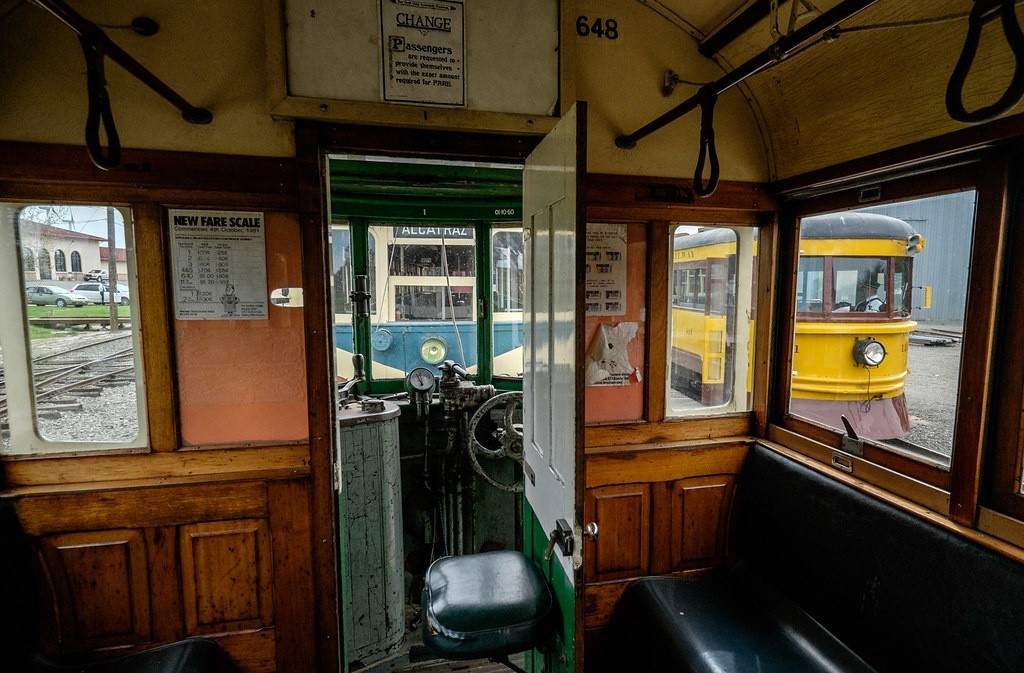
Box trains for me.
[669,213,933,441]
[328,224,528,380]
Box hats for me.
[860,278,881,291]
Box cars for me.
[82,269,109,282]
[25,286,89,308]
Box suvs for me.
[71,282,130,306]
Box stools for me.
[420,548,548,673]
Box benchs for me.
[624,440,1024,673]
[0,494,225,673]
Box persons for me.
[854,284,878,312]
[98,282,107,305]
